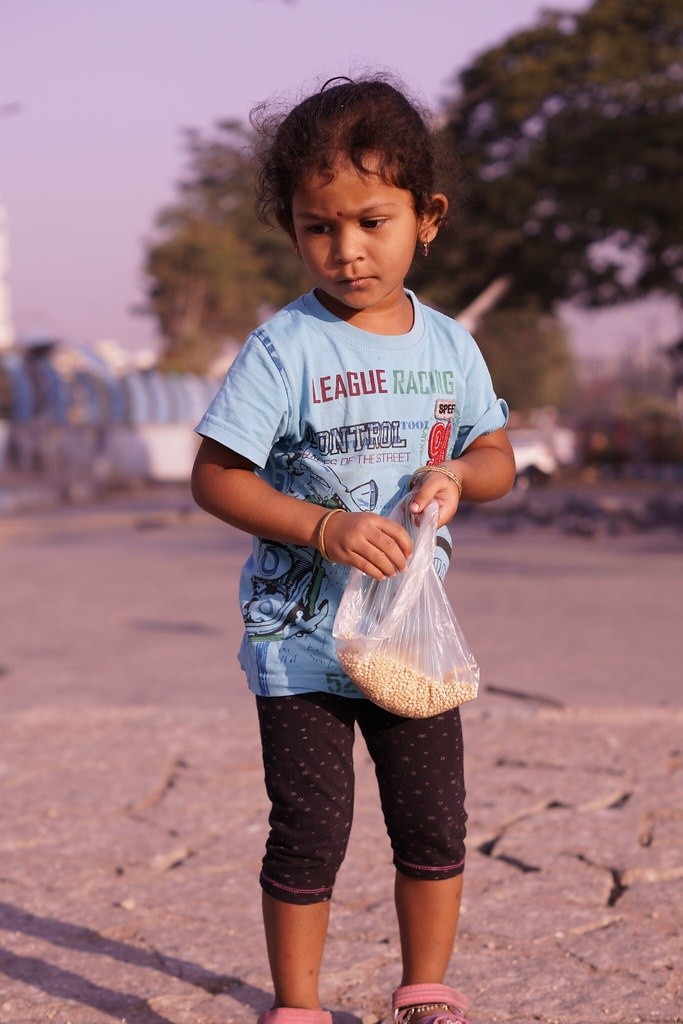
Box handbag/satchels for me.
[332,490,480,719]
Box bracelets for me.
[318,508,346,564]
[407,467,463,496]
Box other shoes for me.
[392,983,471,1024]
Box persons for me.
[190,71,517,1024]
